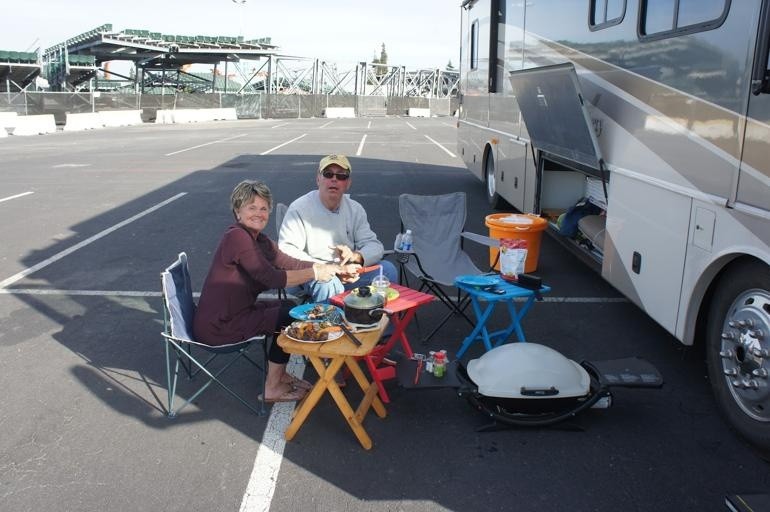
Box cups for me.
[370,275,390,307]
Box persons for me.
[192,180,363,400]
[277,152,398,345]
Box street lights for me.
[232,0,247,7]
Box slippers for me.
[257,384,308,403]
[286,376,312,392]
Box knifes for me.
[355,263,383,274]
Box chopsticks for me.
[338,322,361,346]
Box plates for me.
[455,274,501,287]
[284,319,345,343]
[350,284,400,303]
[287,302,346,322]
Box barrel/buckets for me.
[484,212,549,274]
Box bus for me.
[452,0,769,445]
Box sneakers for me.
[324,359,347,387]
[362,354,398,366]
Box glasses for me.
[322,170,350,181]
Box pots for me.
[341,286,394,325]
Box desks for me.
[327,282,436,406]
[454,274,553,363]
[276,304,390,451]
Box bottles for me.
[397,230,413,263]
[425,350,449,379]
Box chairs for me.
[395,191,504,347]
[158,250,271,422]
[273,201,310,305]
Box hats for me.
[318,153,352,175]
